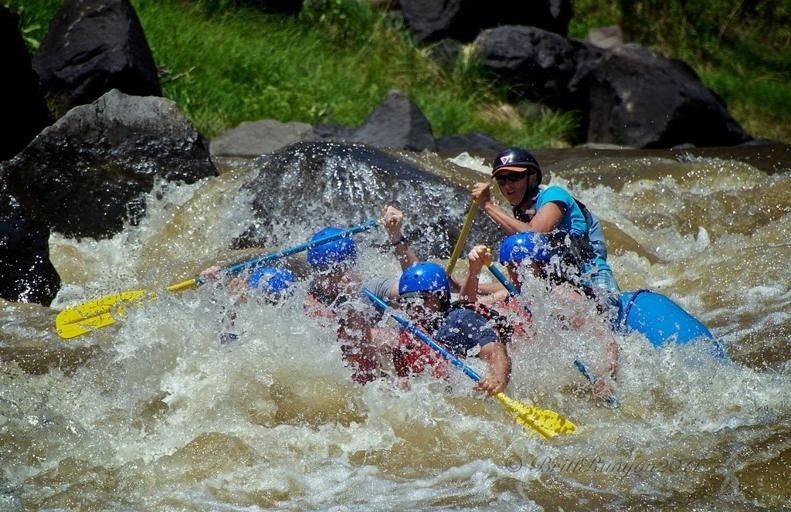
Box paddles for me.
[55,213,402,338]
[362,289,578,442]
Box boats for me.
[617,290,735,397]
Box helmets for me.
[247,265,297,296]
[395,261,452,303]
[499,231,554,266]
[490,147,542,184]
[307,226,358,272]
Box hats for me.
[490,167,528,179]
[396,292,433,302]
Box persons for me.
[198,148,621,402]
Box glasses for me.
[495,171,526,185]
